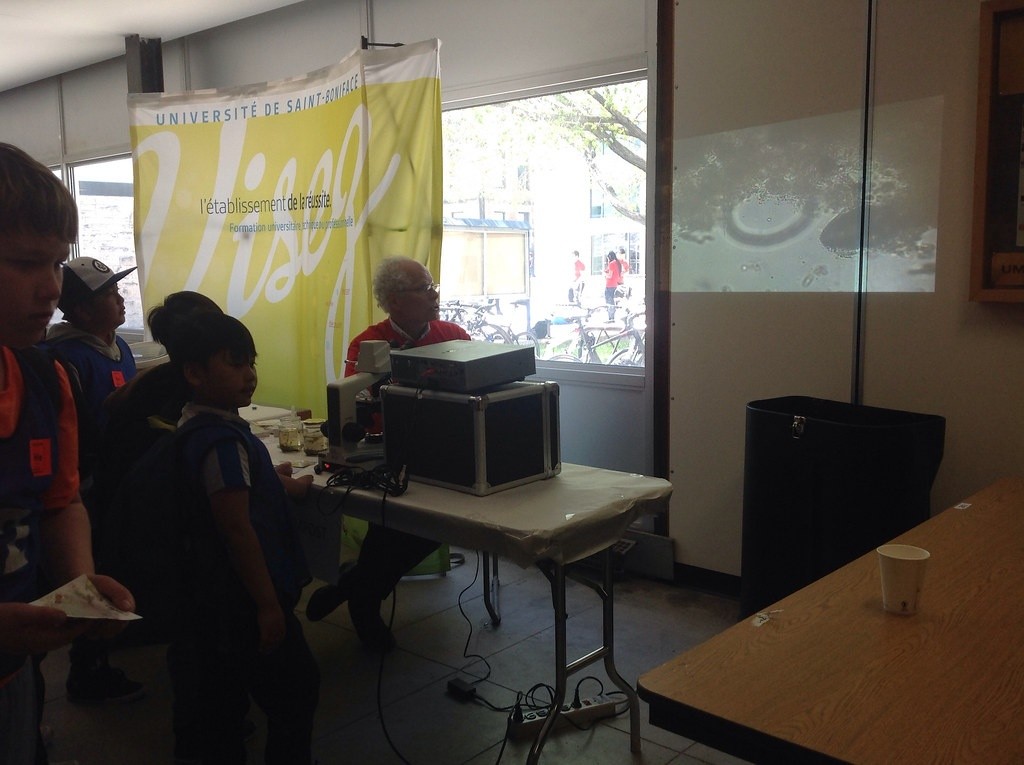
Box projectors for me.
[392,340,537,390]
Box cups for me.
[877,544,930,615]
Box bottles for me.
[278,417,303,452]
[301,418,327,456]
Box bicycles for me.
[440,295,540,358]
[549,305,646,368]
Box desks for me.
[256,418,673,765]
[635,475,1024,765]
[236,402,312,435]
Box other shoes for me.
[64,666,147,706]
[241,720,257,744]
[305,562,347,622]
[346,596,397,654]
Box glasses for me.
[394,282,440,297]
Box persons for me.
[573,251,586,306]
[36,258,320,765]
[0,144,135,765]
[618,249,629,285]
[307,258,471,654]
[604,251,622,322]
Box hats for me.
[58,257,137,323]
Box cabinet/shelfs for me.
[967,0,1024,303]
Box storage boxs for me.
[378,380,562,498]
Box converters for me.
[447,678,477,702]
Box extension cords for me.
[507,695,615,739]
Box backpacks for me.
[67,416,260,657]
